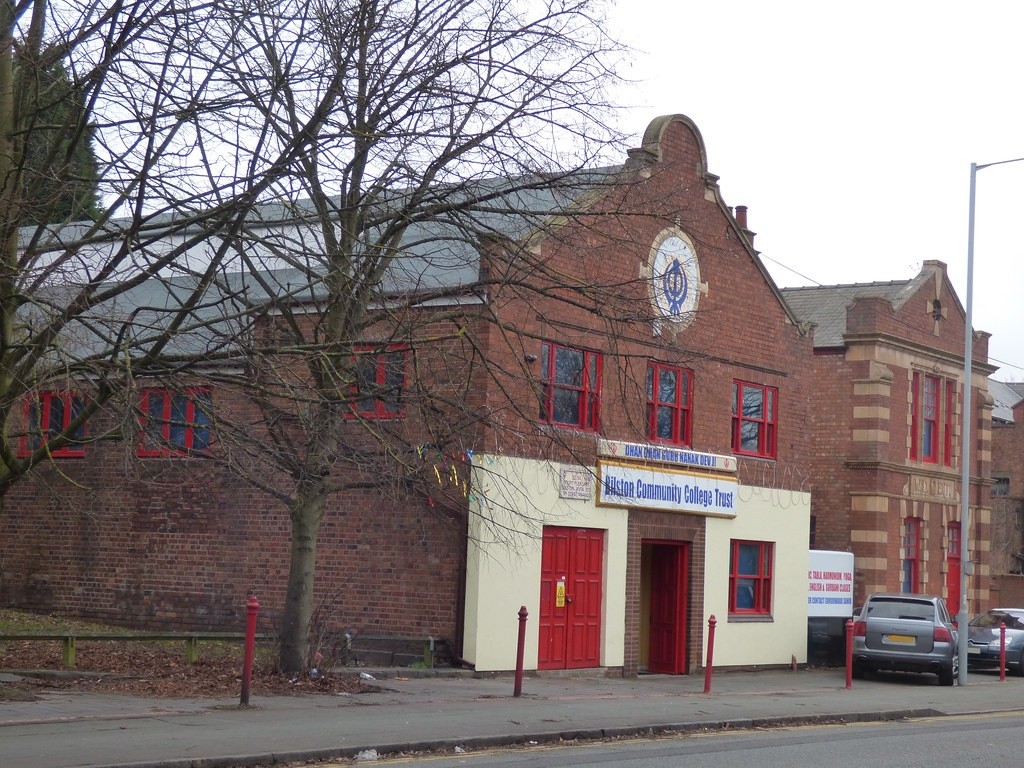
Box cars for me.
[850,593,960,686]
[968,608,1024,675]
